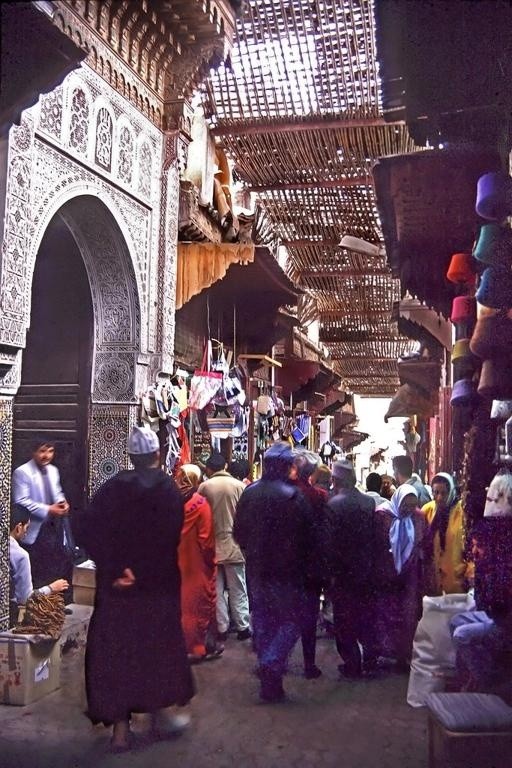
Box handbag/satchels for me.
[188,360,252,441]
[257,393,287,419]
[141,385,187,431]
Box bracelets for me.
[48,584,54,593]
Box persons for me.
[194,451,466,682]
[12,435,76,590]
[10,503,70,603]
[84,426,195,752]
[233,441,310,708]
[451,516,510,692]
[176,463,226,664]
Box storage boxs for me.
[0,642,60,706]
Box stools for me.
[424,691,512,768]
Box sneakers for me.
[207,626,254,655]
[302,664,378,680]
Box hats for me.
[332,459,356,479]
[128,426,160,455]
